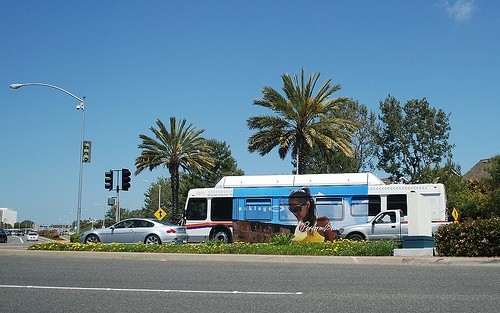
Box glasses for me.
[289,203,305,214]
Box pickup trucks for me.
[339,210,455,242]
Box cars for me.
[17,231,24,236]
[27,231,39,241]
[81,217,188,244]
[0,229,7,243]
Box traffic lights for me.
[104,170,113,191]
[122,169,131,192]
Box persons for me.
[287,187,334,243]
[378,215,395,223]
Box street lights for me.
[10,82,86,232]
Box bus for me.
[182,172,447,245]
